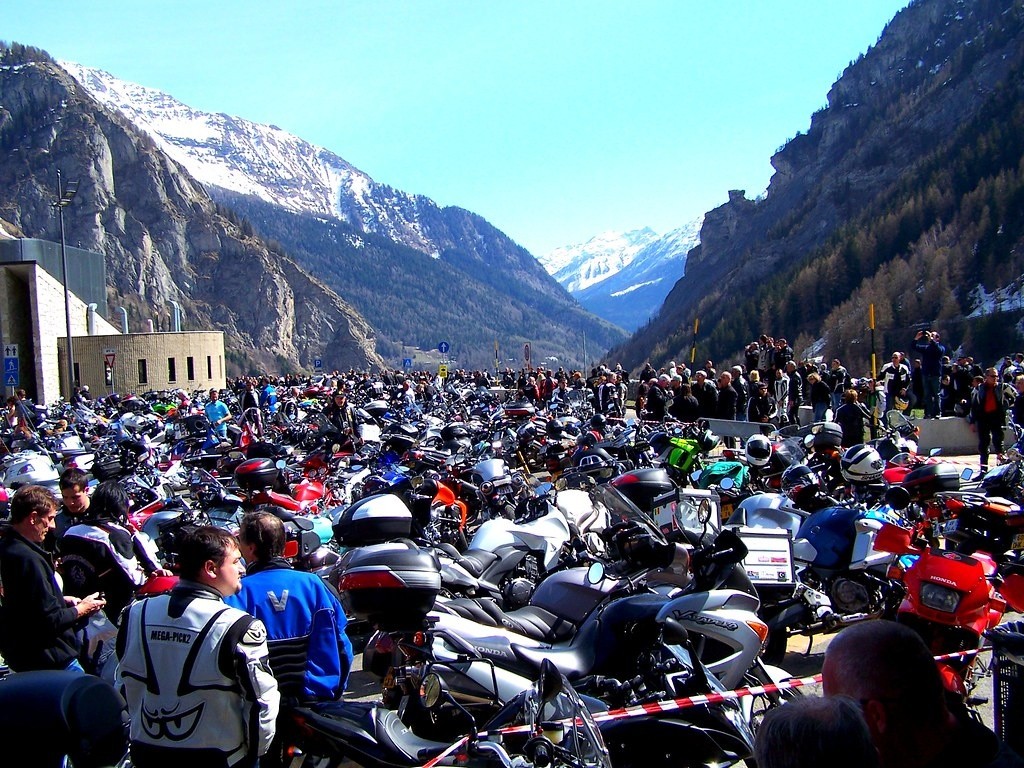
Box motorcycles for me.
[0,376,1024,768]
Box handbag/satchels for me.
[699,461,748,490]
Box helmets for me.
[895,394,908,411]
[840,444,885,484]
[781,463,820,503]
[744,434,772,466]
[546,420,564,438]
[591,414,607,429]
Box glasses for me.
[891,356,899,359]
[988,375,999,378]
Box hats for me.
[333,390,346,399]
[673,375,683,382]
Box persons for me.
[636,331,1024,482]
[222,362,630,418]
[41,468,173,627]
[0,485,106,672]
[753,619,1024,768]
[239,381,260,410]
[7,389,37,429]
[70,385,92,408]
[321,390,364,453]
[224,512,354,768]
[260,377,279,411]
[114,525,281,768]
[205,388,232,436]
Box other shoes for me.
[935,415,941,419]
[924,414,933,419]
[972,470,986,482]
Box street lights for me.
[48,169,83,404]
